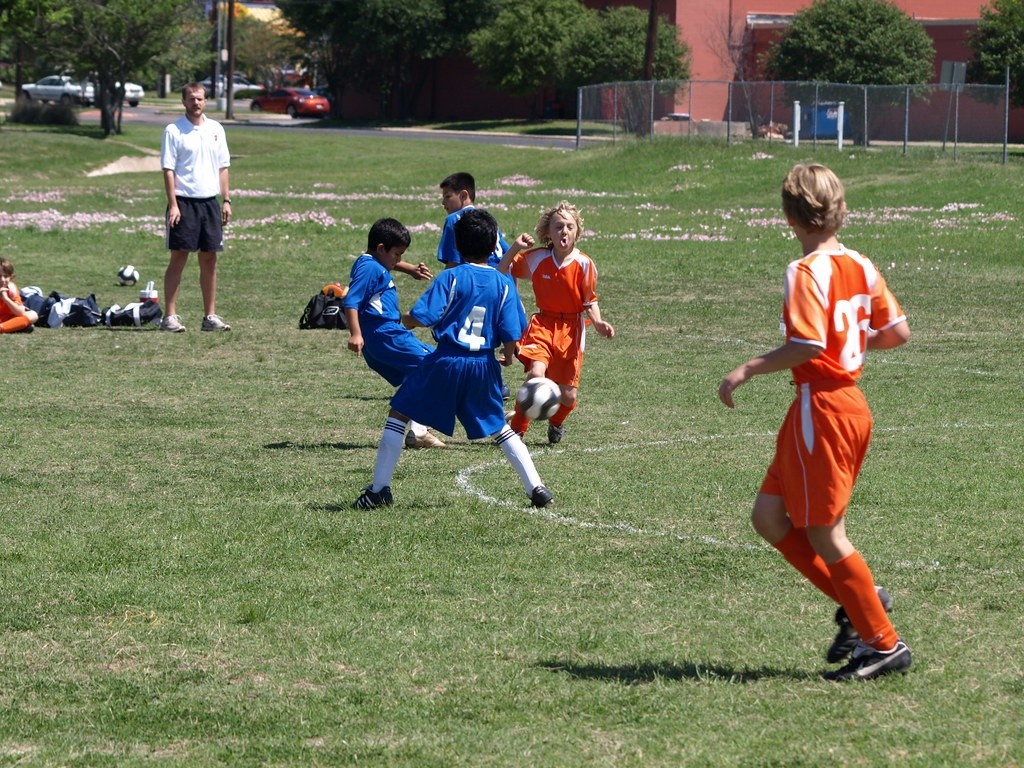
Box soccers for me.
[517,376,562,421]
[117,265,140,286]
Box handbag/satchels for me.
[20,285,102,326]
[298,294,349,329]
[100,300,163,327]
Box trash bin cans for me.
[792,101,851,140]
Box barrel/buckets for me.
[139,281,159,305]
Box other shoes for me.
[502,383,511,399]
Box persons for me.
[349,208,553,512]
[0,257,39,333]
[158,81,232,333]
[497,199,615,443]
[343,217,517,449]
[717,162,912,682]
[437,172,526,400]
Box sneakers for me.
[529,485,555,507]
[548,423,563,444]
[824,642,914,682]
[350,485,393,510]
[201,314,231,331]
[159,315,187,333]
[825,586,893,664]
[504,410,516,419]
[405,430,446,448]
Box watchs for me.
[223,199,231,204]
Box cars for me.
[115,80,145,107]
[21,74,95,106]
[251,86,330,116]
[176,71,264,97]
